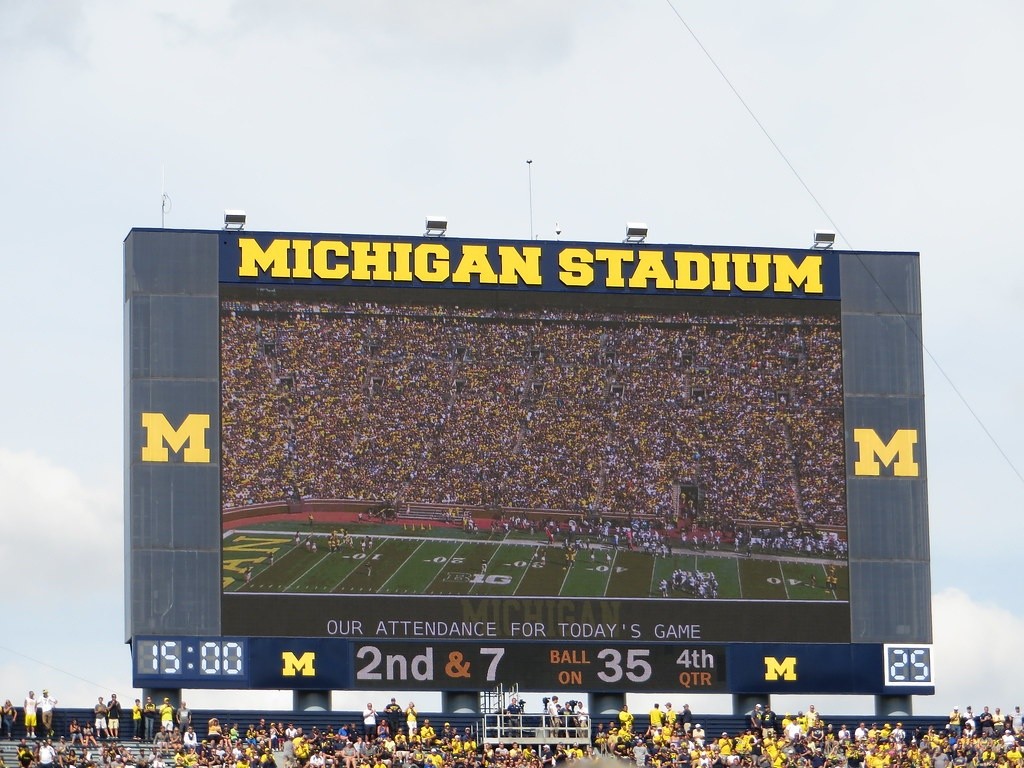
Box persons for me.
[218,299,850,599]
[1,688,1023,768]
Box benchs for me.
[395,505,436,520]
[433,507,471,523]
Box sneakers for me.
[51,729,54,736]
[47,733,49,737]
[31,732,36,739]
[27,732,30,738]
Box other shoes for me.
[112,736,118,738]
[133,736,141,740]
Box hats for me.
[431,749,436,752]
[116,755,121,758]
[445,723,449,726]
[164,698,169,700]
[425,719,429,723]
[465,705,1024,768]
[346,741,353,745]
[43,690,48,694]
[265,748,270,751]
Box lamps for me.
[221,210,247,231]
[622,224,648,244]
[809,231,835,250]
[423,216,448,237]
